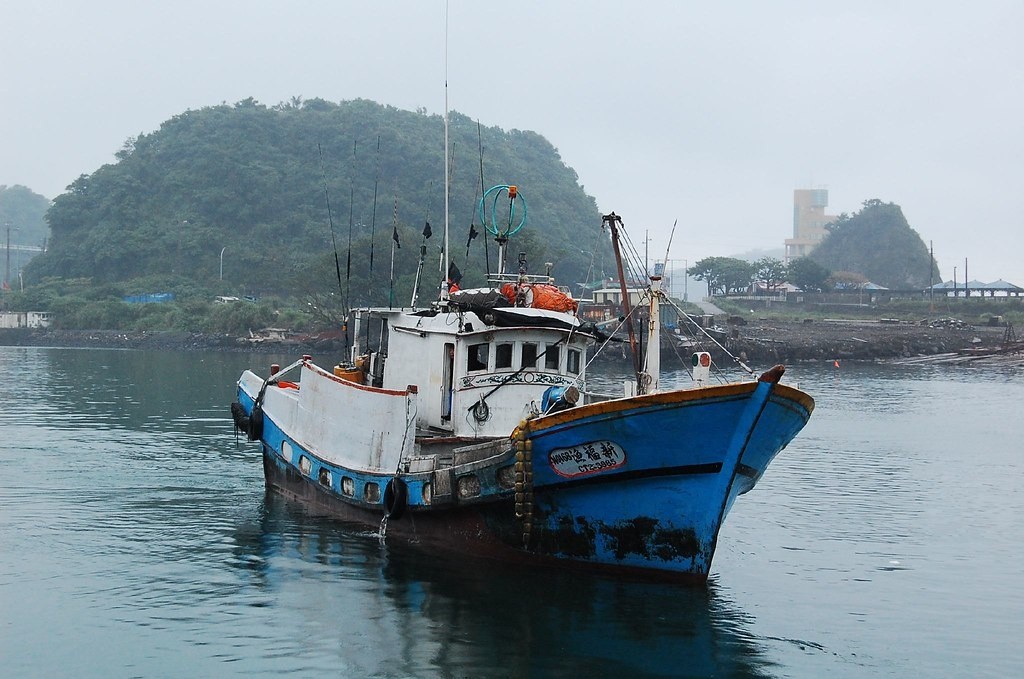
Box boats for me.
[230,0,815,578]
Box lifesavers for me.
[383,478,407,519]
